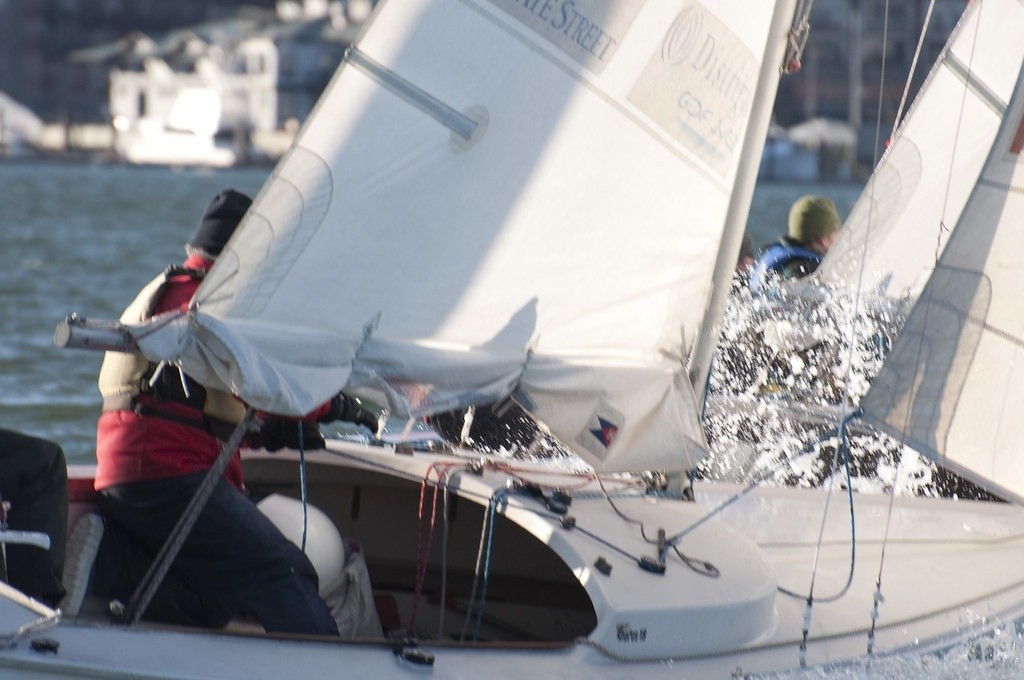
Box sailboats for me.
[1,0,1023,678]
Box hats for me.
[184,187,255,260]
[787,193,844,244]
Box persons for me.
[61,187,380,641]
[752,195,842,292]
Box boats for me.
[759,114,859,184]
